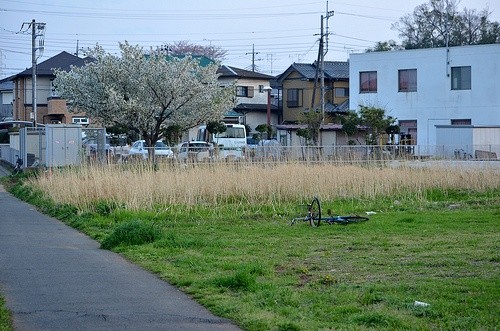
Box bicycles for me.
[290,197,370,228]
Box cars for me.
[83,123,278,165]
